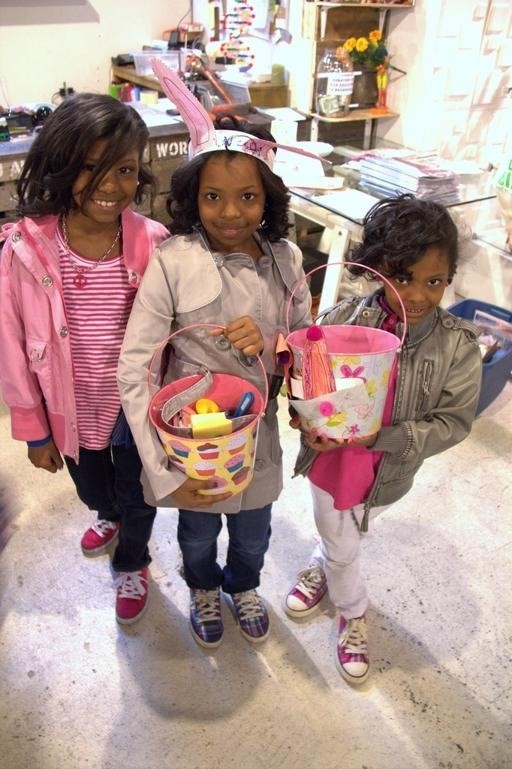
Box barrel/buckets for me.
[283,260,408,441]
[146,321,270,500]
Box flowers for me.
[337,29,391,74]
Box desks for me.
[196,70,289,110]
[272,133,497,325]
[112,64,170,97]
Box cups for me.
[271,64,285,85]
[107,82,159,107]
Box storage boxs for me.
[134,50,180,77]
[0,134,193,242]
[443,298,511,419]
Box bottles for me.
[49,87,75,106]
[315,44,355,119]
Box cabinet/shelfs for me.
[288,0,414,151]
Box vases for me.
[351,64,378,109]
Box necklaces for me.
[62,214,122,290]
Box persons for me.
[116,113,314,650]
[0,93,173,625]
[281,192,483,684]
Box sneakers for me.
[334,612,370,685]
[283,565,328,618]
[81,519,121,555]
[231,588,271,643]
[190,585,224,649]
[115,566,150,625]
